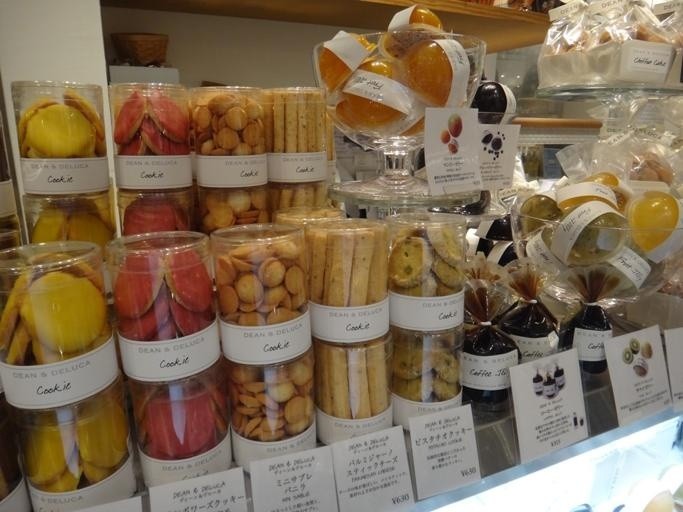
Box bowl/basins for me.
[110,30,167,66]
[506,200,683,307]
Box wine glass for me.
[312,29,487,202]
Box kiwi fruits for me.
[630,338,640,354]
[622,348,633,364]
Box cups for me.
[578,416,584,426]
[476,111,520,125]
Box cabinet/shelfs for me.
[97,0,605,131]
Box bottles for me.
[101,229,237,490]
[8,80,330,243]
[571,410,578,430]
[389,213,463,434]
[1,241,140,512]
[302,217,393,451]
[207,223,318,474]
[0,393,30,511]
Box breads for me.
[632,359,648,376]
[541,18,683,55]
[640,343,653,358]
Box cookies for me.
[0,87,463,500]
[630,151,672,187]
